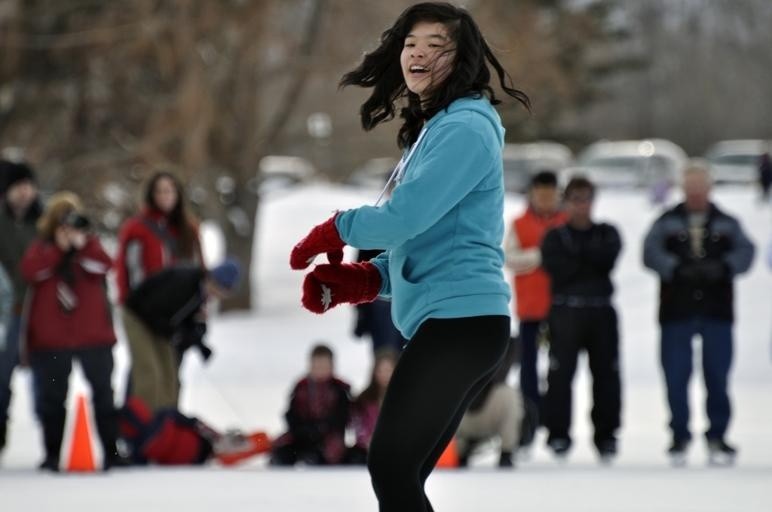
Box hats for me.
[210,263,238,295]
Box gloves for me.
[301,260,383,315]
[290,210,349,270]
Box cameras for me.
[65,209,89,233]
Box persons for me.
[114,173,209,406]
[267,335,523,467]
[642,163,754,456]
[122,263,242,417]
[22,190,145,471]
[289,3,509,512]
[502,171,559,445]
[539,179,624,458]
[352,172,409,351]
[1,146,50,453]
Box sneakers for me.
[670,439,690,453]
[710,438,735,454]
